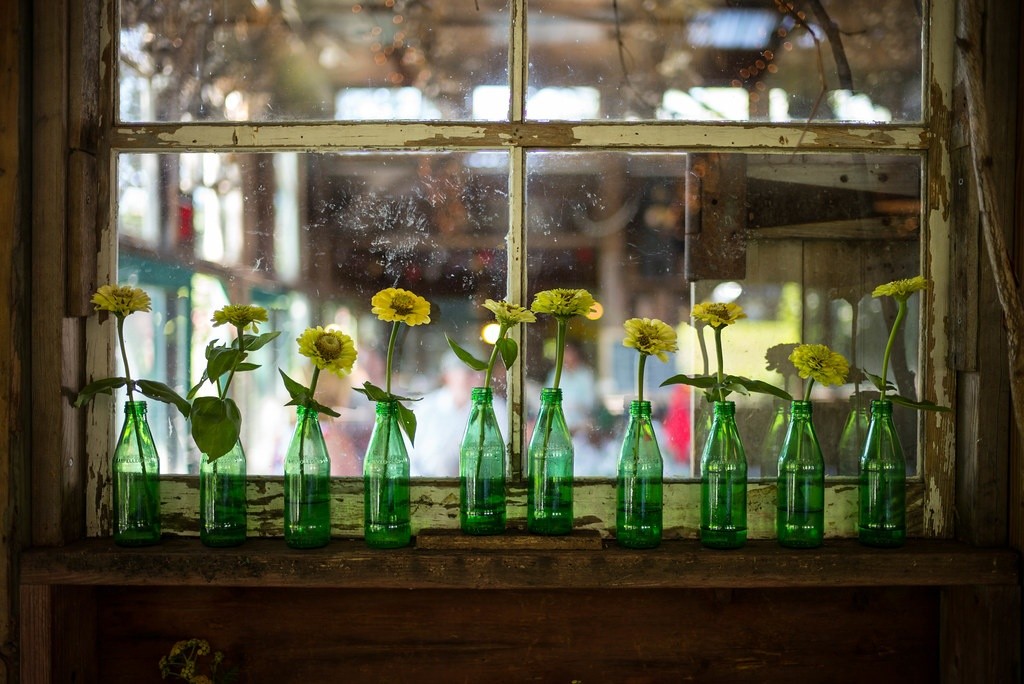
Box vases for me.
[361,402,411,548]
[200,437,247,546]
[459,386,507,537]
[283,404,331,548]
[835,393,870,477]
[694,394,714,478]
[615,399,664,547]
[855,398,906,546]
[774,399,825,549]
[529,387,577,534]
[111,401,161,542]
[761,396,792,478]
[695,399,750,548]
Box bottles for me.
[199,437,248,548]
[699,400,748,551]
[362,401,411,549]
[616,401,663,550]
[759,396,791,479]
[776,400,826,548]
[857,401,908,549]
[837,395,871,477]
[282,404,332,549]
[460,387,507,535]
[111,401,161,540]
[693,395,714,478]
[526,387,574,536]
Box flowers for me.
[615,317,679,528]
[859,276,951,524]
[519,288,597,517]
[774,343,852,527]
[351,287,431,517]
[74,282,191,501]
[764,341,801,435]
[658,302,794,522]
[278,325,358,527]
[186,303,281,530]
[442,297,537,517]
